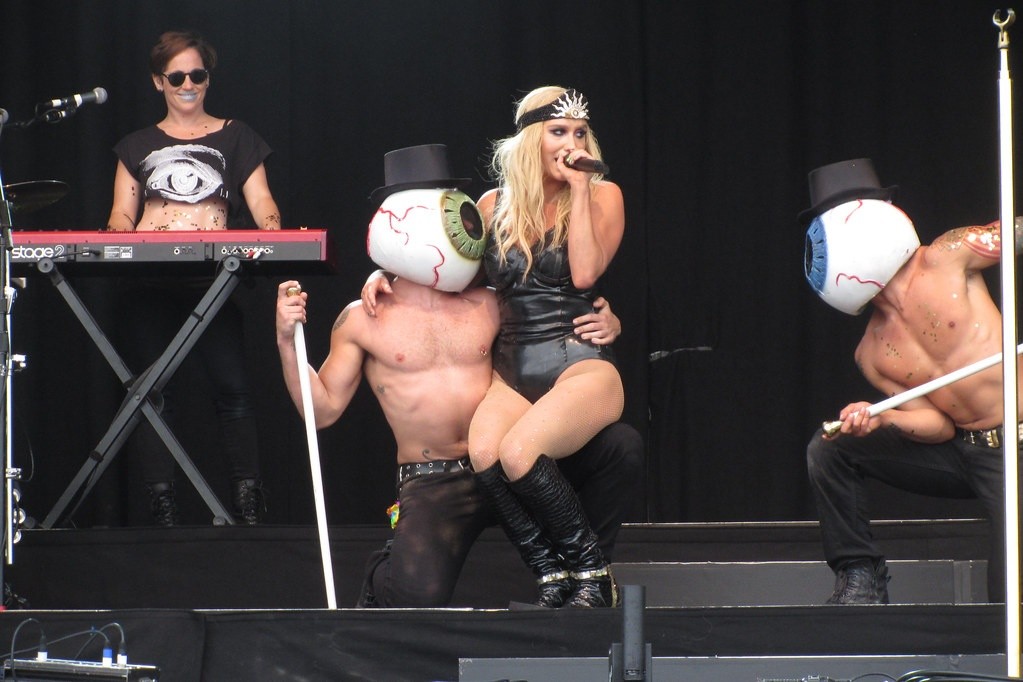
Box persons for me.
[799,156,1023,608]
[275,86,645,609]
[107,29,282,522]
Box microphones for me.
[45,87,107,108]
[0,108,9,125]
[563,153,604,174]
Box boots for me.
[473,454,621,609]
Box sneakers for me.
[821,562,891,605]
[228,477,269,528]
[148,483,180,527]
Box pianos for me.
[4,226,329,527]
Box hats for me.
[368,143,476,201]
[796,156,901,225]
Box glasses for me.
[160,68,210,87]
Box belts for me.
[956,422,1023,449]
[395,458,471,482]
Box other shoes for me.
[355,550,387,609]
